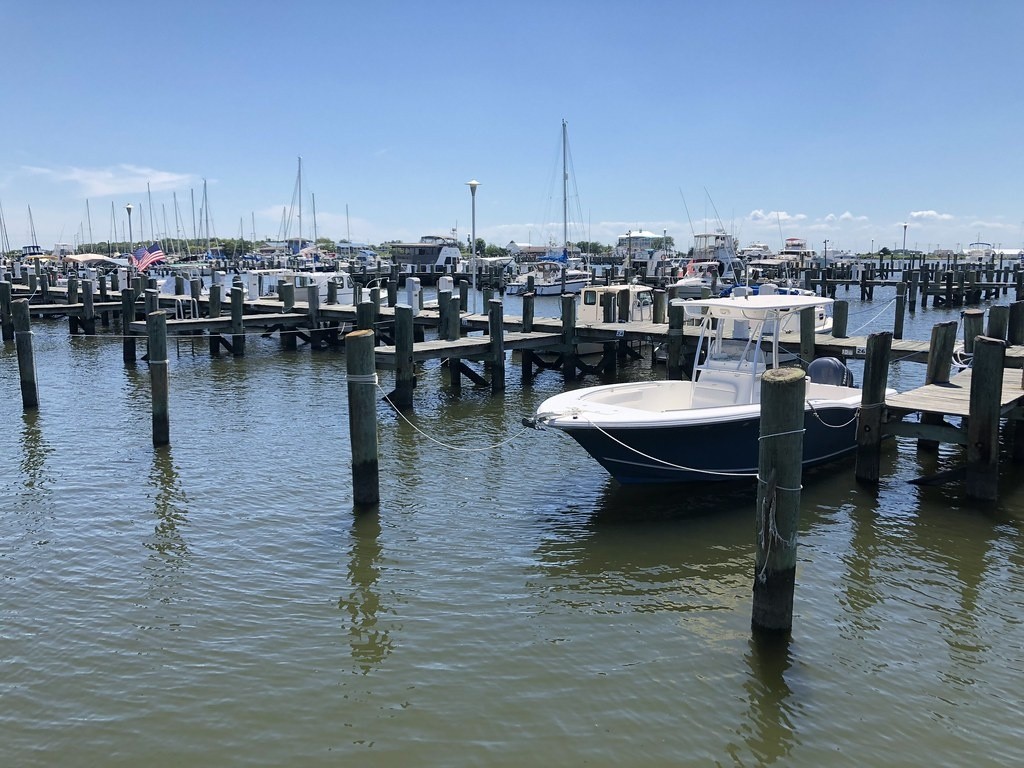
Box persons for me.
[699,267,704,273]
[682,265,688,276]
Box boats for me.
[967,242,992,262]
[522,293,902,497]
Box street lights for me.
[901,222,908,281]
[662,229,667,256]
[822,239,829,268]
[465,179,482,315]
[871,239,874,259]
[124,203,134,254]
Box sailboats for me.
[0,119,859,337]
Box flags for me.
[127,242,166,273]
